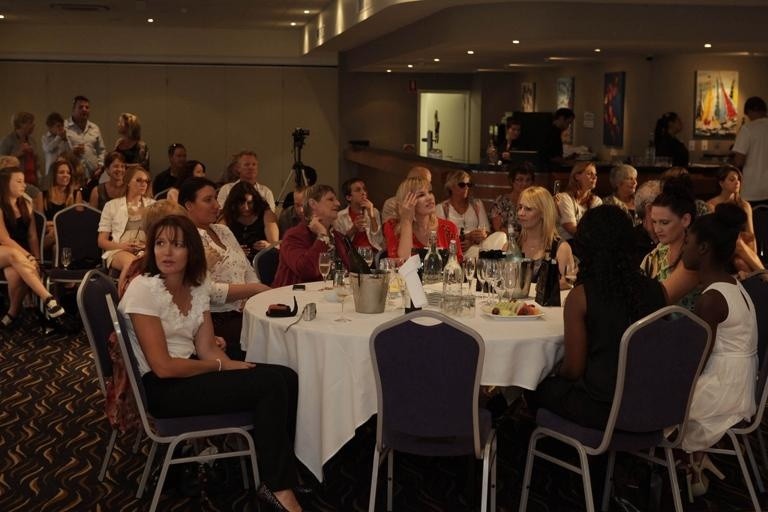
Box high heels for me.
[1,311,22,330]
[42,296,66,322]
[685,452,726,496]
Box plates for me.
[481,301,545,319]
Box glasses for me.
[457,182,472,189]
[135,177,148,184]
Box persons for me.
[3,97,767,512]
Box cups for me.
[437,295,476,317]
[380,259,403,308]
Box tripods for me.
[275,142,310,207]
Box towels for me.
[397,253,427,308]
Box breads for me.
[268,303,288,315]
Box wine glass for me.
[490,260,497,303]
[463,256,476,297]
[504,262,519,301]
[333,270,352,322]
[482,260,496,299]
[477,259,487,297]
[358,246,373,268]
[494,261,506,303]
[319,253,331,290]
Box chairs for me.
[253,237,280,284]
[369,309,497,512]
[0,210,45,336]
[641,368,767,512]
[153,189,168,199]
[47,203,107,334]
[76,271,146,480]
[103,294,260,510]
[520,306,708,512]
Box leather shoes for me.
[256,479,306,512]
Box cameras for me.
[554,180,561,195]
[294,128,310,137]
[303,303,317,321]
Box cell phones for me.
[292,284,305,290]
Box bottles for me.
[489,140,497,162]
[646,129,656,165]
[423,231,443,285]
[502,227,520,255]
[443,240,462,306]
